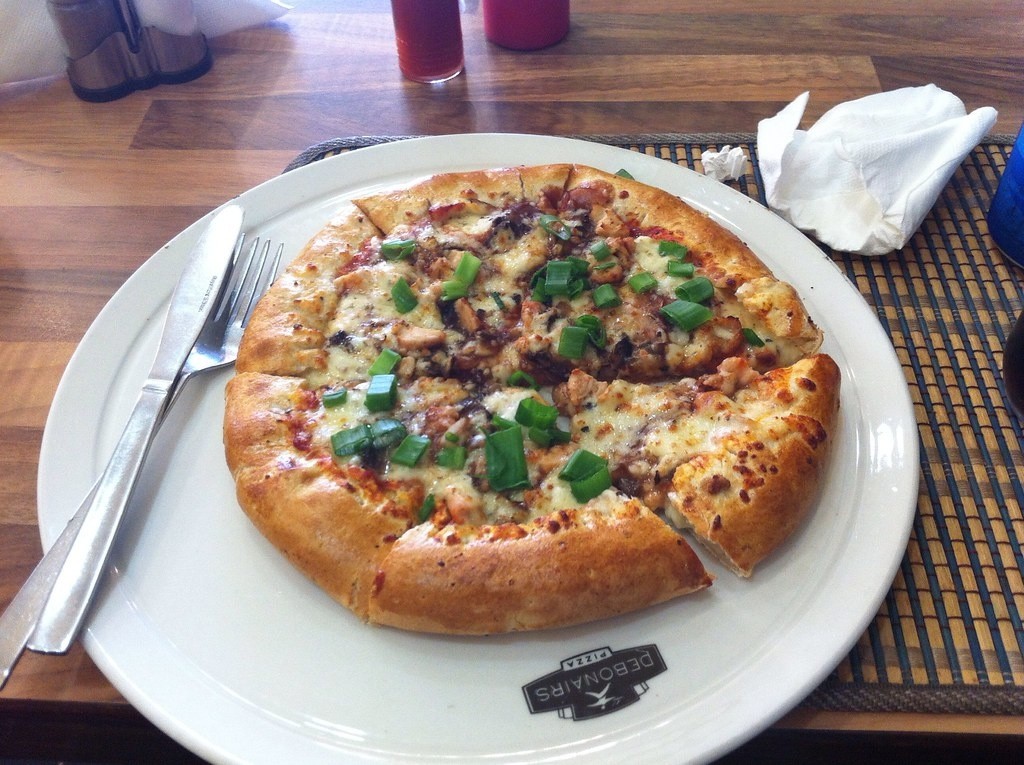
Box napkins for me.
[755,81,998,255]
[0,0,295,85]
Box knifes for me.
[26,204,243,655]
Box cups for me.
[987,124,1024,269]
[391,0,465,84]
[483,0,569,49]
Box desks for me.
[0,0,1024,765]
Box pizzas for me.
[224,161,840,636]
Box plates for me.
[39,132,919,764]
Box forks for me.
[0,233,282,687]
